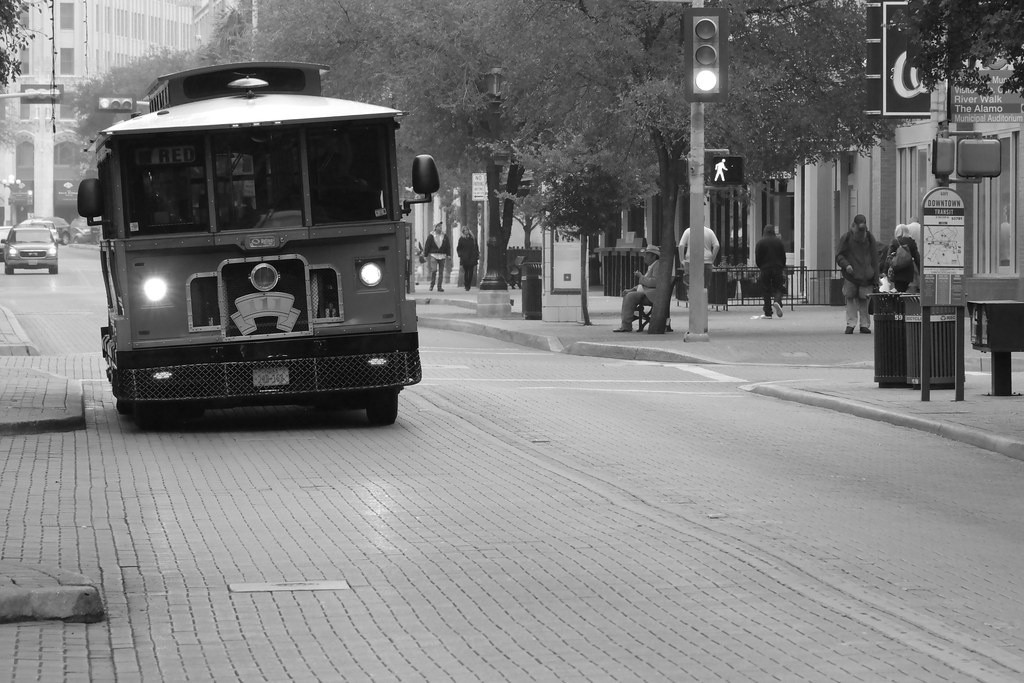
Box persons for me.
[125,173,189,223]
[424,221,451,291]
[612,245,660,331]
[457,226,479,291]
[414,238,424,284]
[907,216,921,289]
[679,226,720,289]
[835,214,880,334]
[882,224,920,295]
[755,224,786,318]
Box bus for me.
[78,61,440,426]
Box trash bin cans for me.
[519,262,542,320]
[899,294,955,390]
[867,292,911,388]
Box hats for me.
[894,224,910,240]
[854,214,866,228]
[433,221,443,230]
[641,245,660,257]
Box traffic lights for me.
[711,155,744,187]
[683,8,728,103]
[94,94,137,113]
[932,138,955,179]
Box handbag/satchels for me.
[419,249,426,263]
[868,286,879,315]
[890,237,912,271]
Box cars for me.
[1,228,63,275]
[0,226,13,261]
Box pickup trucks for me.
[22,219,71,245]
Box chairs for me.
[636,275,680,334]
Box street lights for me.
[476,67,512,318]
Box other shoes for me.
[761,315,772,319]
[845,327,854,334]
[860,327,871,334]
[774,302,783,317]
[429,286,433,291]
[437,287,444,292]
[613,328,632,332]
[632,315,639,323]
